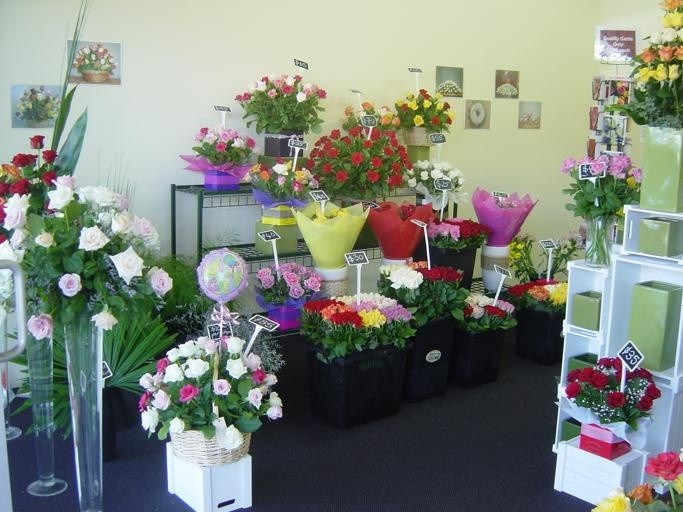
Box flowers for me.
[342,100,402,133]
[511,236,533,266]
[567,355,654,425]
[513,276,573,306]
[392,85,454,136]
[140,335,283,447]
[398,156,467,199]
[257,261,321,301]
[236,72,330,112]
[431,214,486,245]
[249,157,321,197]
[378,258,455,304]
[3,135,65,241]
[193,124,254,167]
[630,1,682,89]
[4,175,174,341]
[295,202,364,256]
[311,125,411,189]
[462,294,512,327]
[370,201,427,247]
[592,449,682,511]
[304,289,414,341]
[563,151,643,189]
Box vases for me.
[583,217,615,268]
[168,427,253,465]
[18,296,69,501]
[63,331,107,512]
[320,267,349,297]
